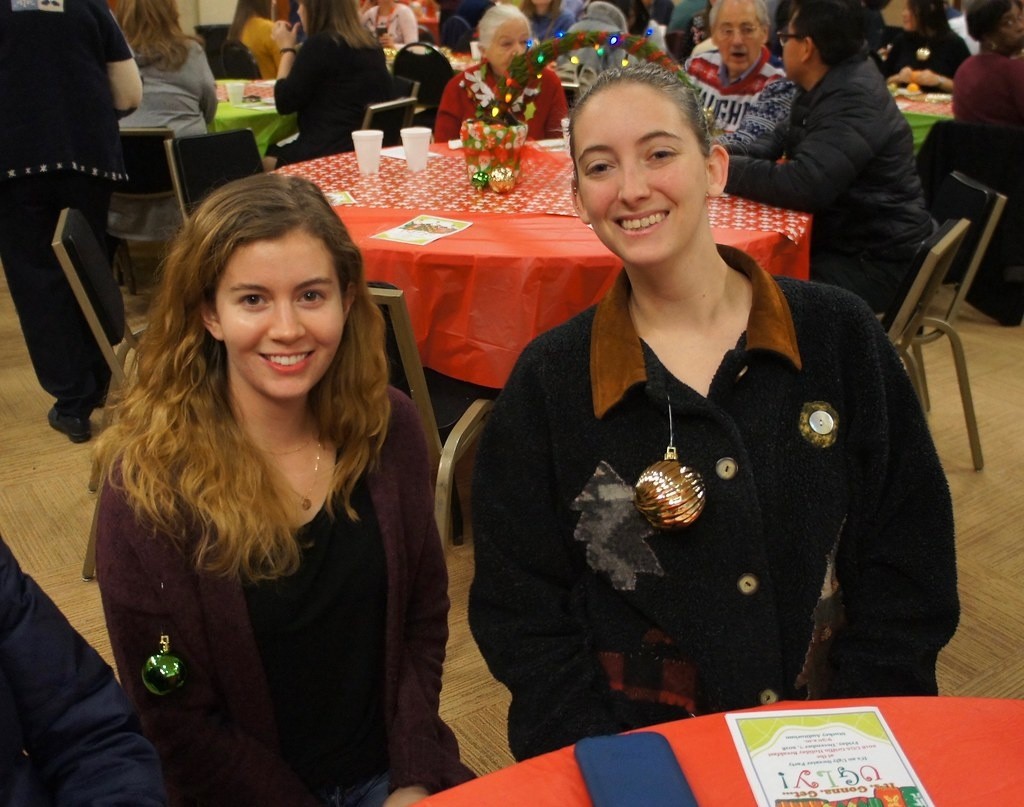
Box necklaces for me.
[261,433,322,511]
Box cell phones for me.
[573,731,699,807]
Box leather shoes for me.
[48,406,93,442]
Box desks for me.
[201,77,299,174]
[416,696,1024,807]
[260,132,812,390]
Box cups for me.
[561,118,572,152]
[400,126,432,170]
[352,129,384,173]
[224,83,245,105]
[470,41,481,60]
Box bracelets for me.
[280,48,297,55]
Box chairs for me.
[47,43,1008,549]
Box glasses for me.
[777,30,799,47]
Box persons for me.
[232,0,1024,329]
[104,0,218,280]
[467,68,962,767]
[0,0,143,447]
[0,533,168,807]
[92,169,479,807]
